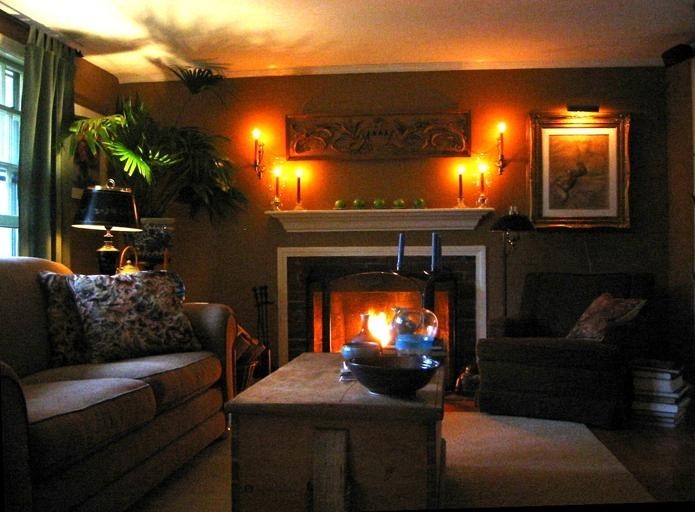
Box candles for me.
[456,162,488,197]
[253,128,261,165]
[274,167,303,204]
[498,122,506,158]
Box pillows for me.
[36,266,200,368]
[564,291,648,342]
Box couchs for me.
[0,256,238,512]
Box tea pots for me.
[114,245,140,275]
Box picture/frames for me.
[526,110,632,230]
[284,110,473,160]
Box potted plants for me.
[69,63,248,257]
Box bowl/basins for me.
[342,353,441,399]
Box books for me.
[627,358,692,428]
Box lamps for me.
[70,176,146,275]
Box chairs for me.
[477,271,638,424]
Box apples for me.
[353,198,365,207]
[373,199,385,208]
[414,199,424,208]
[393,199,404,207]
[335,200,345,208]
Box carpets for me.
[122,403,660,509]
